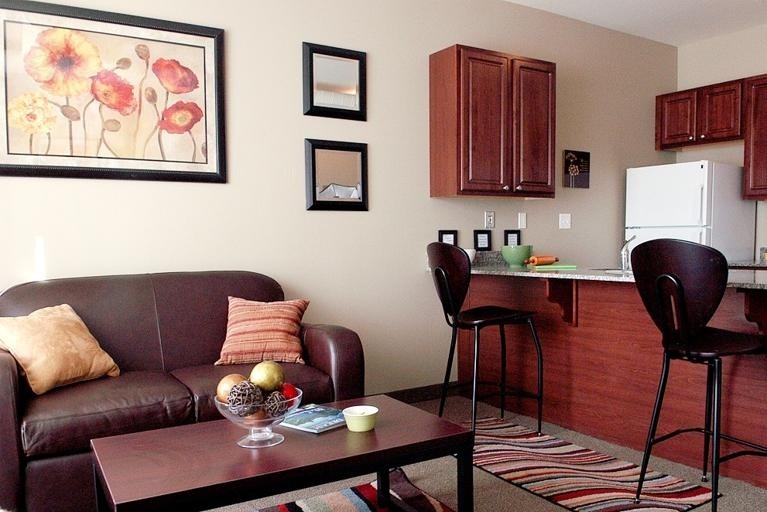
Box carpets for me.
[258,466,455,512]
[448,415,722,510]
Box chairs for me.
[630,239,766,511]
[426,241,543,436]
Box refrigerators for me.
[624,160,757,266]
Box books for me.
[279,402,347,434]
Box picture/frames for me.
[303,43,370,211]
[0,1,228,184]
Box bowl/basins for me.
[463,249,478,262]
[500,244,533,268]
[342,403,381,435]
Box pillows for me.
[213,292,313,367]
[0,302,120,398]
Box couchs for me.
[0,271,365,511]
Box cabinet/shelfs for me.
[737,73,766,201]
[654,80,740,150]
[427,43,555,197]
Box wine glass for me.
[211,387,303,450]
